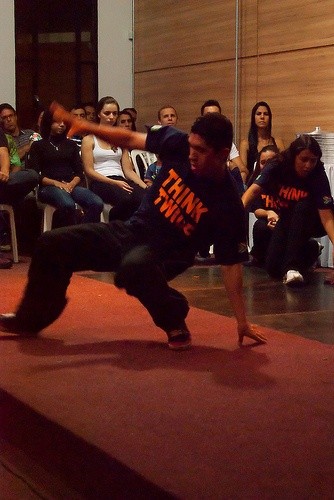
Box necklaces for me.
[48,139,63,151]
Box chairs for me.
[0,173,113,263]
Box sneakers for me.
[286,269,304,285]
[0,317,39,338]
[164,322,193,350]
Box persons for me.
[80,101,99,125]
[81,96,150,223]
[196,99,249,262]
[111,111,150,183]
[122,107,138,132]
[0,102,42,169]
[0,132,24,252]
[66,104,90,159]
[0,101,269,350]
[242,134,334,285]
[157,105,178,127]
[244,145,280,266]
[0,130,41,269]
[26,105,105,230]
[241,101,286,191]
[27,104,47,136]
[250,193,334,278]
